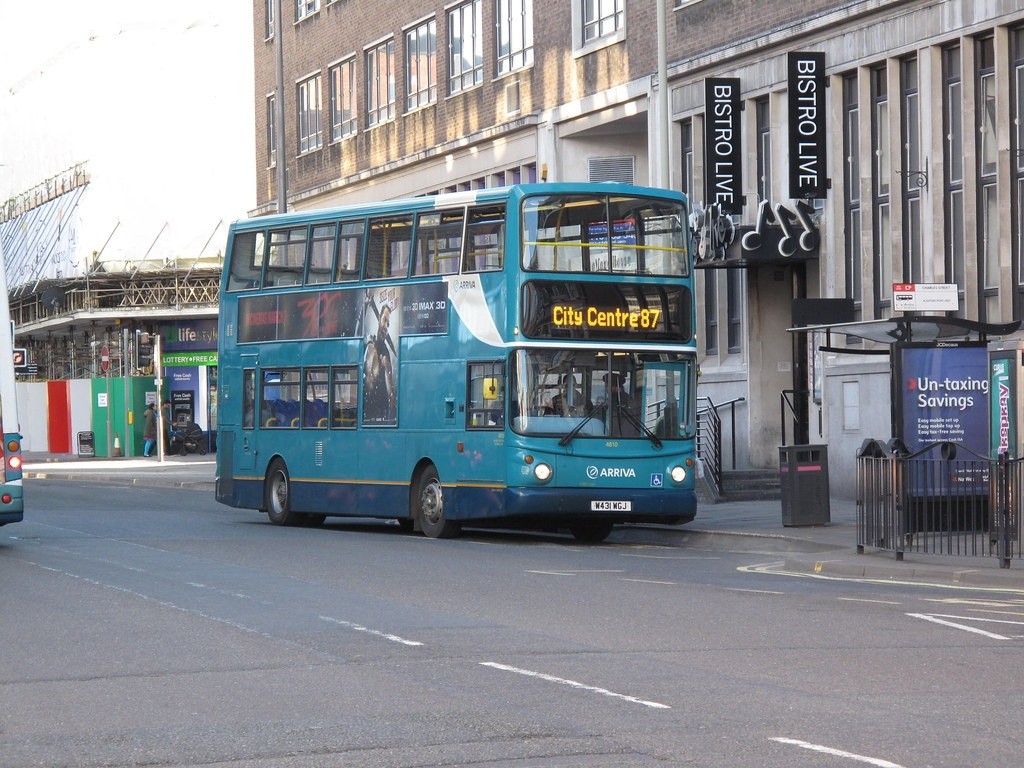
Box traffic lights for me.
[135,329,152,372]
[11,349,28,369]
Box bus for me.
[214,180,701,546]
[0,246,25,528]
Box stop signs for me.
[100,345,111,371]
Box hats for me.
[603,373,626,386]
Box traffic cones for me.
[112,432,124,457]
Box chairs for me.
[247,397,341,427]
[247,267,364,287]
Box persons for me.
[544,375,585,416]
[143,403,156,456]
[161,400,178,455]
[653,416,664,437]
[364,304,394,422]
[586,373,634,438]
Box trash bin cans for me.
[777,443,830,529]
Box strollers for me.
[169,421,208,456]
